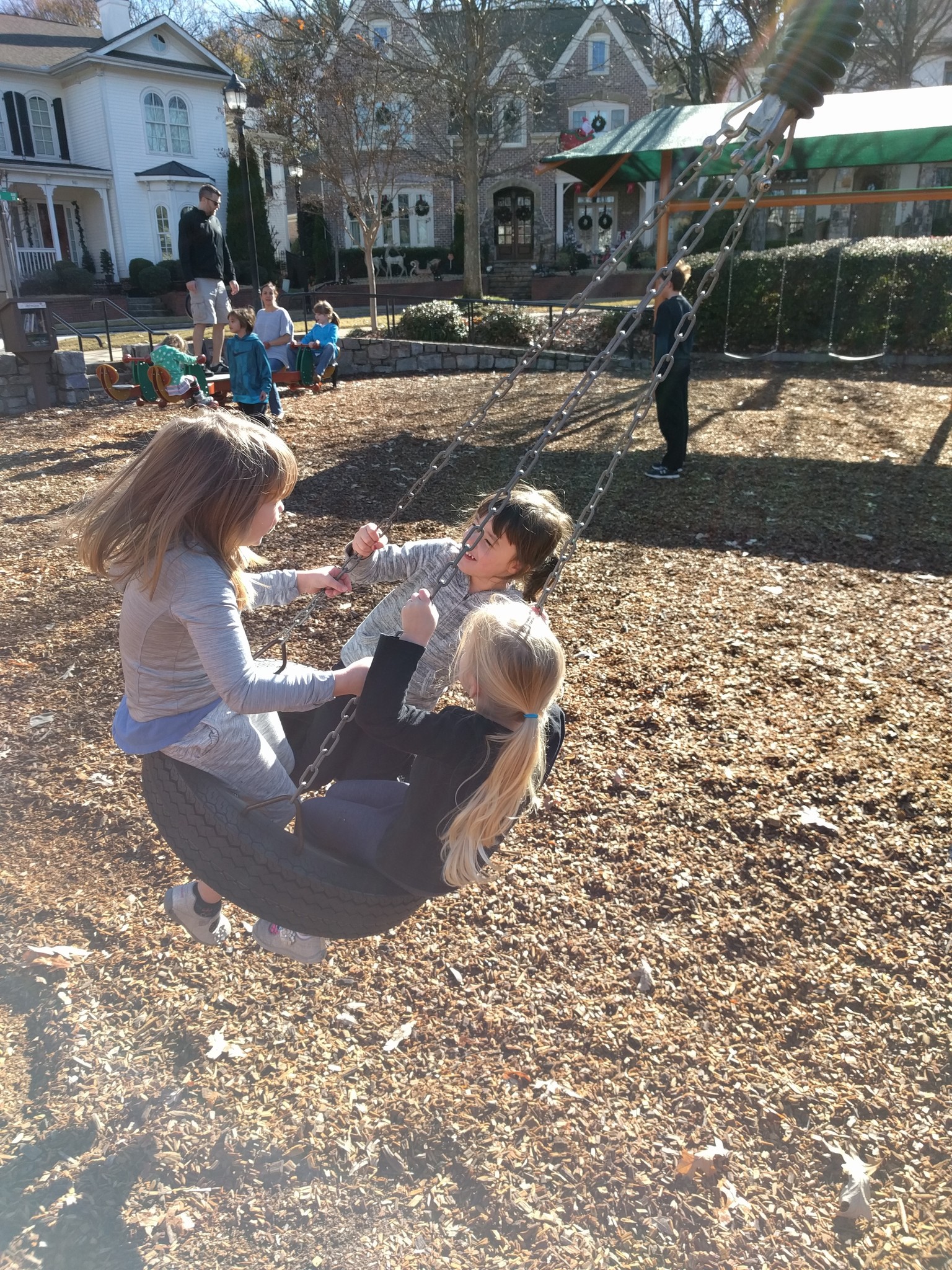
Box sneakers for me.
[200,364,214,377]
[210,361,229,374]
[644,462,683,479]
[252,917,328,964]
[163,882,231,945]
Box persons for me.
[225,308,276,431]
[151,333,213,407]
[597,244,617,264]
[64,407,297,946]
[246,282,295,421]
[295,479,570,787]
[642,266,698,480]
[178,183,240,378]
[252,592,567,966]
[619,230,627,241]
[286,300,341,391]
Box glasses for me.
[205,197,221,207]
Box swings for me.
[130,83,797,932]
[721,208,789,362]
[825,197,903,361]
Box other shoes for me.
[271,411,284,420]
[283,381,297,384]
[312,373,321,383]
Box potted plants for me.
[99,249,116,284]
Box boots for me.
[192,390,214,407]
[184,397,195,409]
